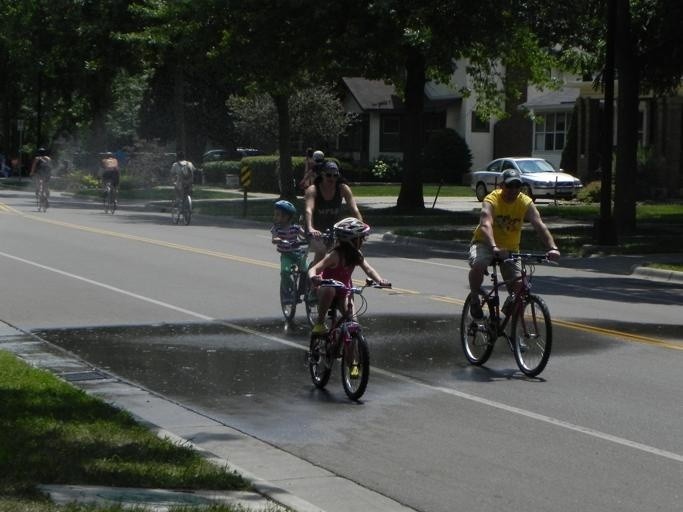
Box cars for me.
[471,157,583,202]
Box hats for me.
[498,169,524,184]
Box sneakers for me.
[282,291,294,304]
[311,321,330,335]
[465,317,489,336]
[351,363,360,380]
[510,336,529,352]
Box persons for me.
[28,148,52,195]
[99,151,121,203]
[169,151,196,214]
[271,200,309,298]
[274,149,282,197]
[467,168,561,352]
[307,217,392,379]
[303,148,315,195]
[298,150,325,185]
[303,159,364,270]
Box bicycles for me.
[102,178,118,214]
[170,182,192,225]
[272,229,369,328]
[30,173,50,212]
[310,279,391,400]
[461,251,558,377]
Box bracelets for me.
[491,245,497,249]
[550,246,559,250]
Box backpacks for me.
[177,160,192,184]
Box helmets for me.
[313,150,324,160]
[332,216,372,241]
[273,200,297,216]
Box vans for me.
[201,149,264,162]
